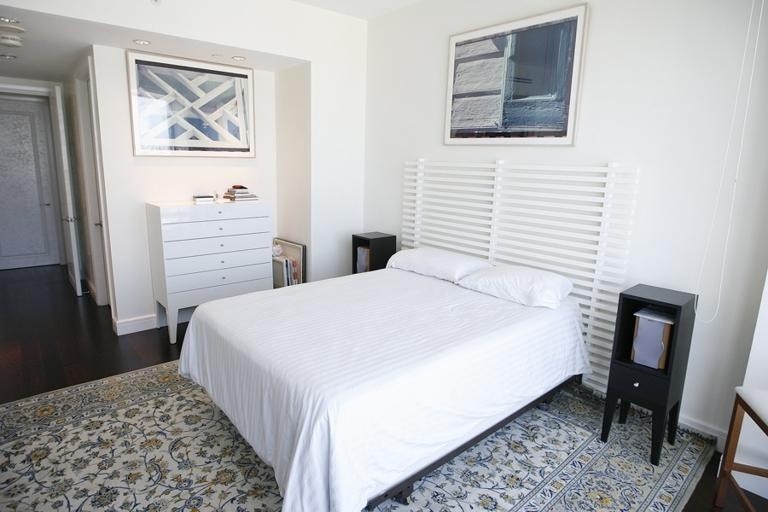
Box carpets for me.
[1,356,717,511]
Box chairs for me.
[710,383,767,511]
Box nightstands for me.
[350,230,398,274]
[600,283,699,465]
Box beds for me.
[177,155,634,511]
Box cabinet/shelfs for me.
[143,195,276,346]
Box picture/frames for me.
[442,3,591,148]
[124,48,260,161]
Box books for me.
[357,246,370,273]
[224,185,259,202]
[193,191,218,204]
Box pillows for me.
[455,259,576,309]
[386,241,492,284]
